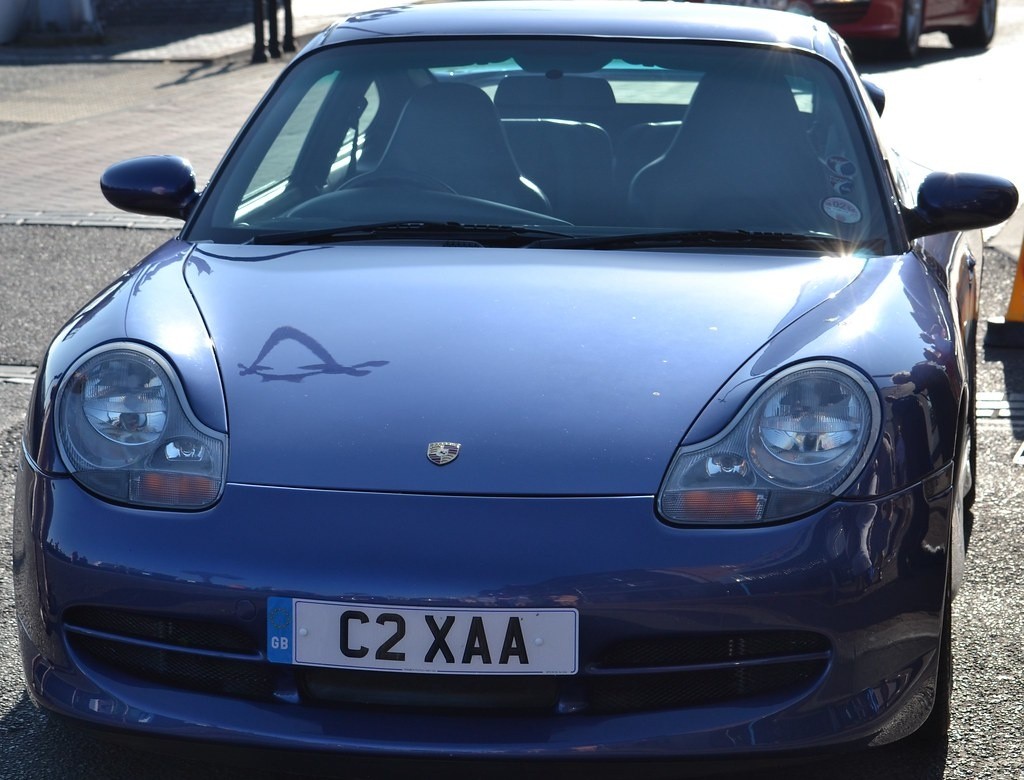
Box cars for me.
[795,1,1002,63]
[8,0,1023,780]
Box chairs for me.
[378,69,854,238]
